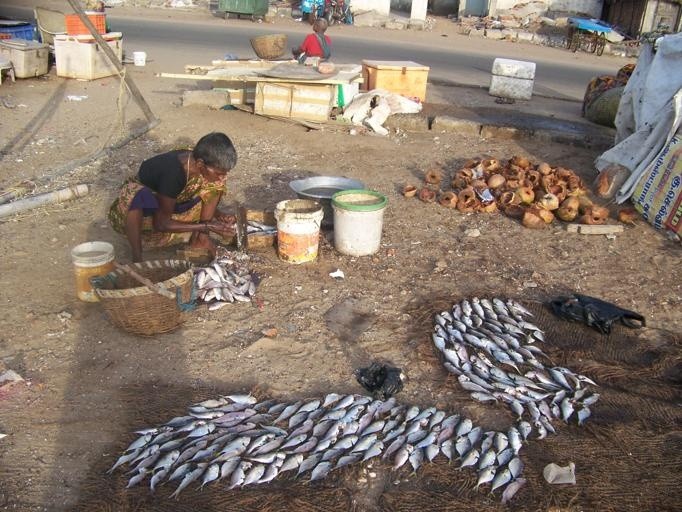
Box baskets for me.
[250,34,288,59]
[88,259,195,338]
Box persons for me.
[108,133,237,263]
[292,17,332,64]
[93,2,111,32]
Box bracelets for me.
[205,222,208,234]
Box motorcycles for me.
[325,0,354,26]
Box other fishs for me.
[107,392,528,506]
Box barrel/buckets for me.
[330,190,388,257]
[273,198,324,264]
[133,51,146,67]
[70,241,115,303]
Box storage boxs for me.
[0,20,37,40]
